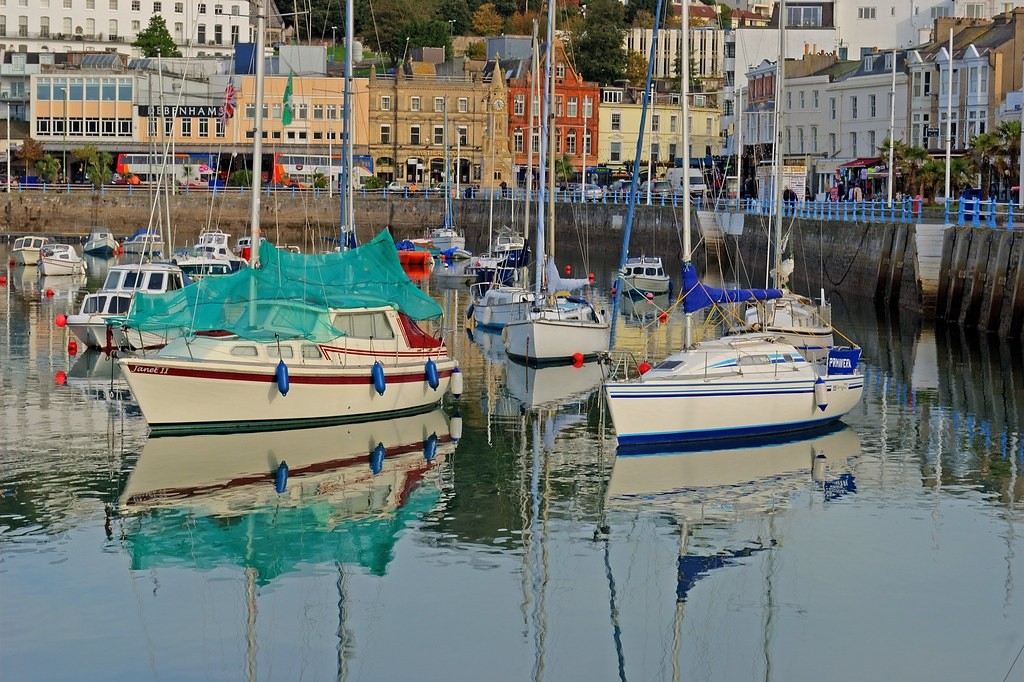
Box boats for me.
[84,228,118,257]
[64,218,192,349]
[37,243,88,276]
[122,228,165,254]
[10,235,56,266]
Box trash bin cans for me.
[959,188,982,219]
[441,185,449,197]
[21,176,38,190]
[210,179,224,190]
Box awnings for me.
[867,169,903,177]
[839,157,882,167]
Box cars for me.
[570,184,603,203]
[387,182,419,198]
[433,182,462,191]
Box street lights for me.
[59,88,68,182]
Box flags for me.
[218,76,237,124]
[282,67,294,125]
[828,349,862,374]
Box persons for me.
[409,182,416,196]
[913,192,923,217]
[500,180,507,197]
[4,176,18,185]
[824,180,862,202]
[111,173,143,184]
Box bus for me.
[116,152,226,191]
[516,165,611,194]
[271,152,374,191]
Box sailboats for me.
[604,0,867,454]
[403,94,471,260]
[725,0,836,345]
[466,0,609,368]
[611,81,671,297]
[112,1,460,442]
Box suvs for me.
[611,179,674,202]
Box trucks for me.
[666,167,706,198]
